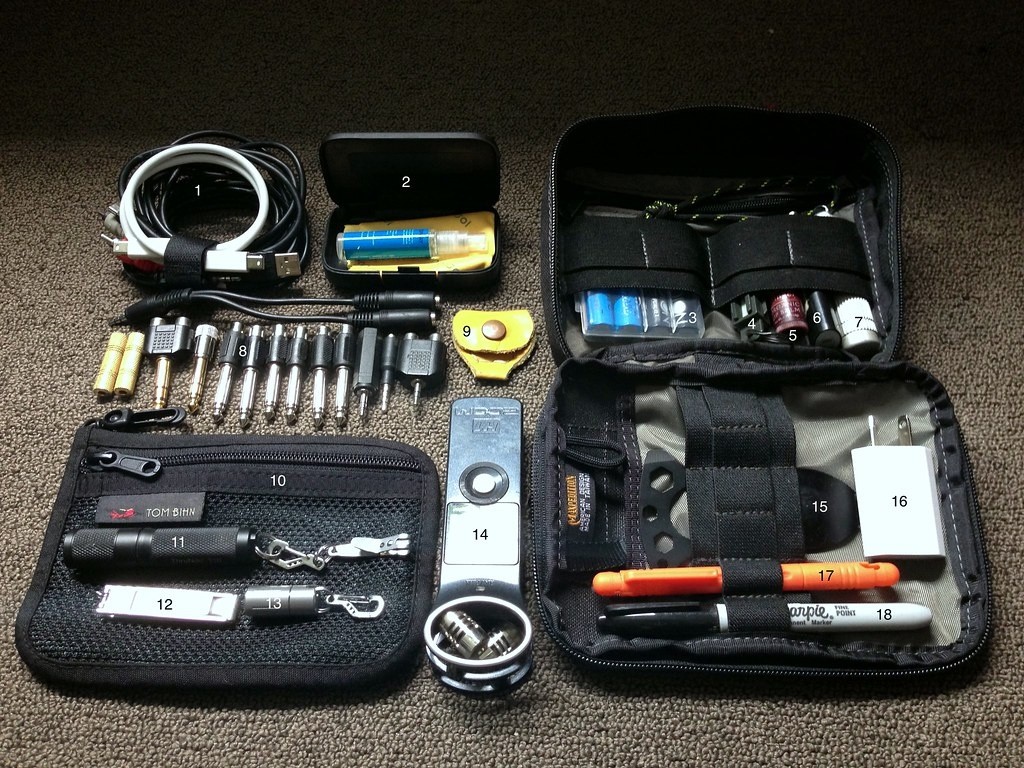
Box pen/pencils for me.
[599,600,933,638]
[591,561,900,597]
